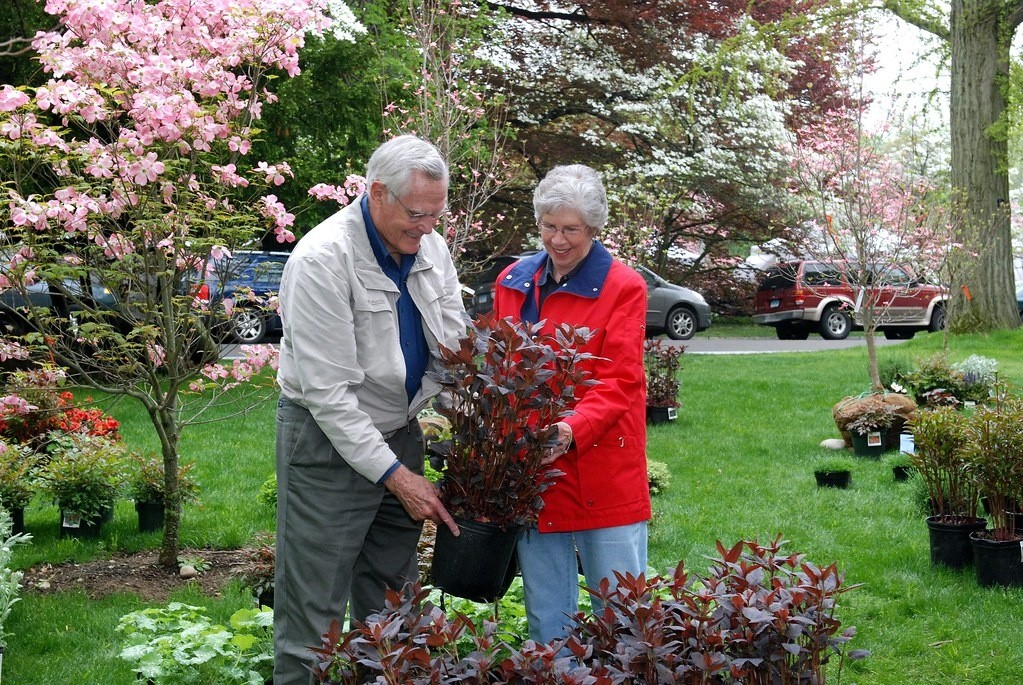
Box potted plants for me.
[427,310,613,604]
[49,427,144,538]
[0,437,48,542]
[908,371,1023,587]
[832,388,917,455]
[133,465,165,532]
[643,337,689,425]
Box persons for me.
[272,135,470,685]
[480,163,652,669]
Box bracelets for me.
[563,425,572,454]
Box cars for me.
[1012,261,1023,303]
[0,262,117,321]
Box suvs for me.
[176,250,292,343]
[466,249,712,341]
[751,258,951,340]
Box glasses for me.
[387,186,450,219]
[535,220,589,235]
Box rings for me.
[551,448,553,455]
